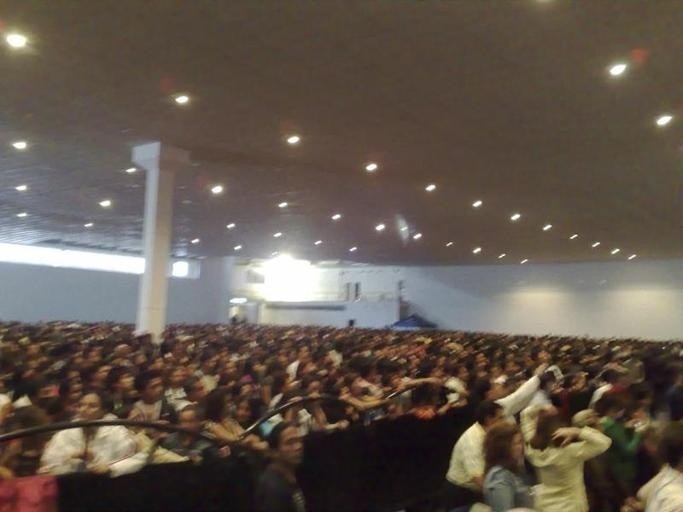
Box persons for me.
[230,314,238,322]
